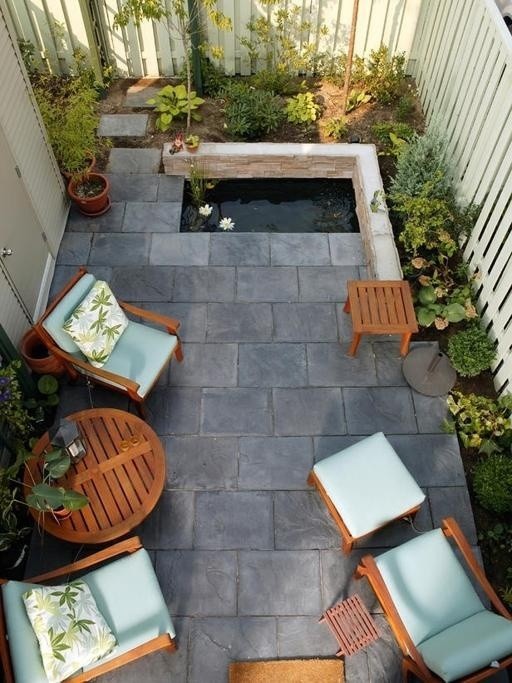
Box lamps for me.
[46,417,87,466]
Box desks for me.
[20,406,167,546]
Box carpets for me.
[225,658,346,683]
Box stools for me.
[344,275,418,359]
[320,594,381,661]
[308,429,427,555]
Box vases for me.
[19,331,67,378]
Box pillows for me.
[20,578,117,682]
[59,278,130,368]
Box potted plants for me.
[5,447,90,534]
[28,70,111,218]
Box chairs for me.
[0,537,177,683]
[30,267,187,421]
[352,514,510,683]
